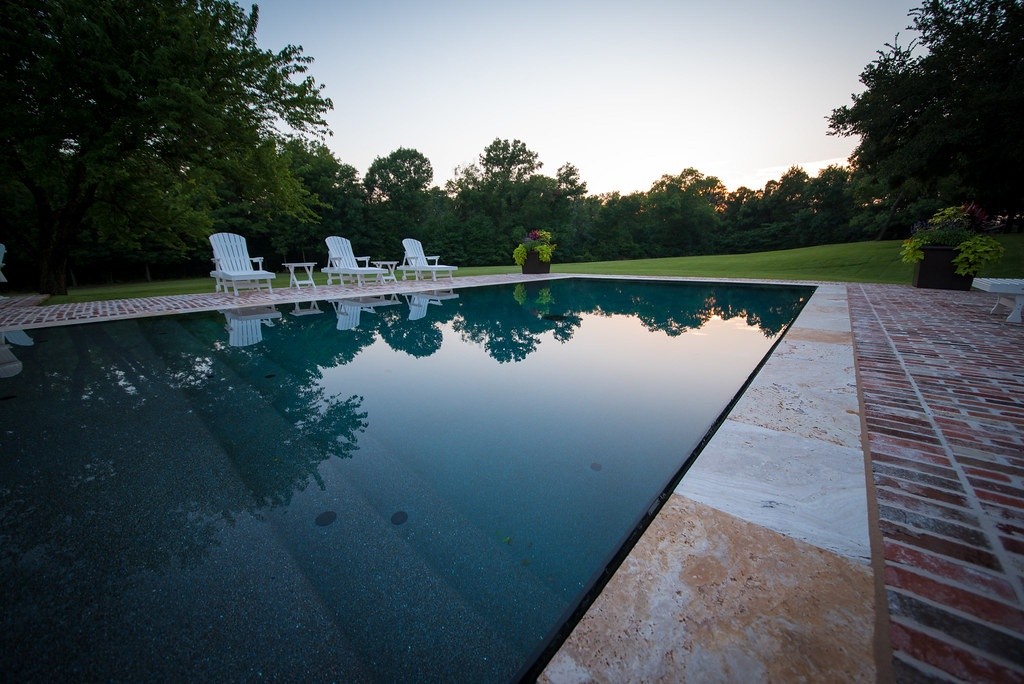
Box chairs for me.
[321,236,388,287]
[400,288,460,320]
[209,233,276,297]
[326,294,386,331]
[217,304,282,347]
[397,238,458,281]
[971,277,1024,323]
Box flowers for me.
[513,284,556,320]
[513,229,557,266]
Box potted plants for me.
[900,205,1005,291]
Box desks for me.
[281,262,318,289]
[382,293,403,305]
[371,261,399,285]
[289,301,325,317]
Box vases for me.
[521,251,550,274]
[521,280,551,289]
